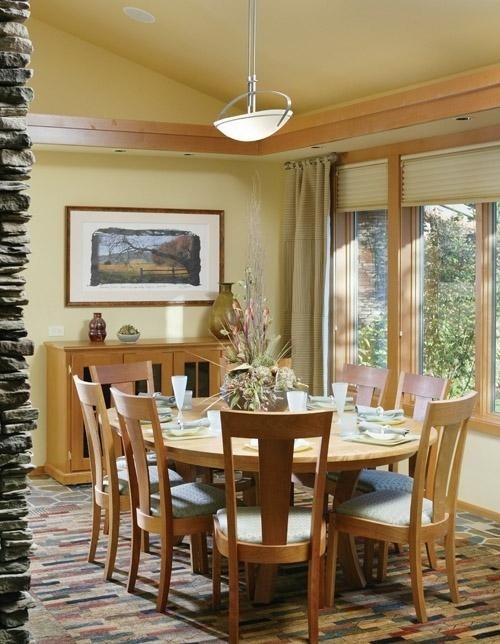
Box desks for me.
[97,396,439,589]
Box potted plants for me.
[116,324,140,342]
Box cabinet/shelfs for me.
[44,337,234,482]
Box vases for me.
[209,278,244,340]
[89,312,108,341]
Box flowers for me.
[181,168,310,417]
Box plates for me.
[368,434,396,441]
[139,406,173,423]
[243,438,310,454]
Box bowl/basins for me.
[116,332,141,344]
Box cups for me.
[205,410,221,434]
[181,390,193,411]
[286,390,309,412]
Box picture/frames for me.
[65,203,226,309]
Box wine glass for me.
[331,381,349,424]
[171,375,188,432]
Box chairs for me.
[216,356,295,397]
[110,385,254,613]
[326,362,390,495]
[73,374,198,582]
[89,359,156,533]
[333,370,451,576]
[327,389,478,624]
[210,409,333,644]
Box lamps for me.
[210,0,296,142]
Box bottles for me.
[88,312,106,343]
